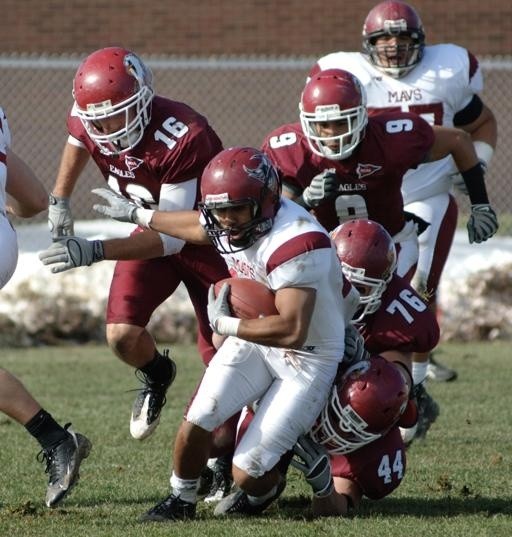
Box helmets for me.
[309,356,410,458]
[298,68,369,162]
[330,219,398,324]
[197,146,281,255]
[361,1,425,79]
[70,47,154,158]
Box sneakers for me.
[135,457,287,521]
[414,386,439,436]
[427,357,457,382]
[36,423,92,508]
[129,357,176,440]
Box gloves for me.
[302,171,334,208]
[92,188,156,230]
[206,282,242,337]
[467,203,499,244]
[343,325,370,362]
[39,237,105,274]
[290,433,334,500]
[47,193,75,236]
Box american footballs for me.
[212,277,278,320]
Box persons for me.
[39,45,225,442]
[0,104,91,506]
[235,355,408,517]
[260,68,500,375]
[90,145,360,522]
[328,219,441,451]
[302,1,498,437]
